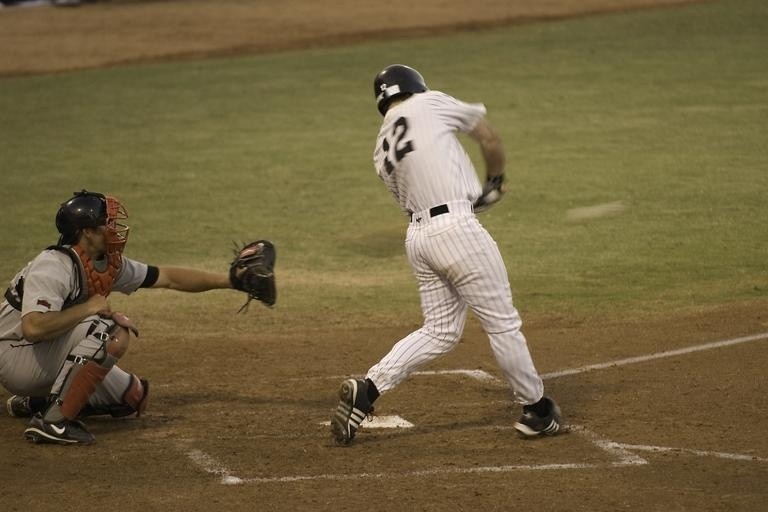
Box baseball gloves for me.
[230,240,276,306]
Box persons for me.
[329,63,570,445]
[0,187,278,447]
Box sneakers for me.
[25,413,96,445]
[513,398,563,438]
[6,396,38,418]
[332,376,375,445]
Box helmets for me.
[373,63,427,112]
[54,192,111,235]
